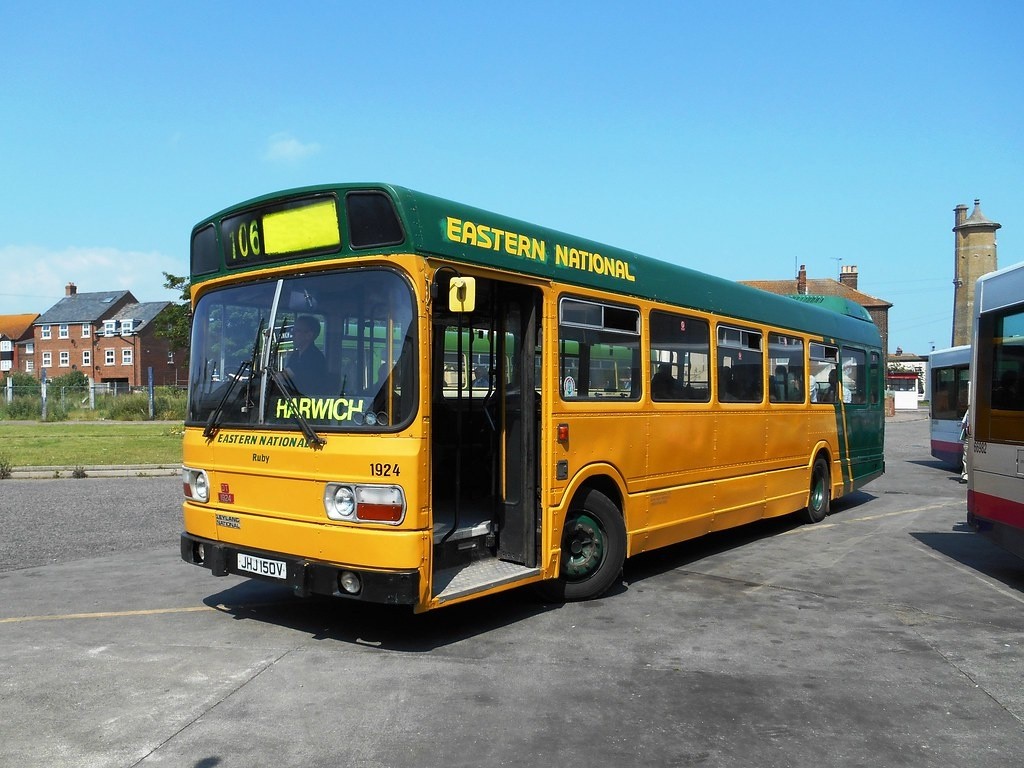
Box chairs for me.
[992,369,1020,410]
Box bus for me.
[180,184,886,615]
[927,335,1023,466]
[969,260,1024,538]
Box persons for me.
[959,409,968,483]
[473,368,489,386]
[654,365,675,397]
[229,316,324,394]
[771,359,817,402]
[822,370,852,402]
[723,366,739,400]
[365,362,400,397]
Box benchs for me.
[438,405,496,456]
[850,389,875,404]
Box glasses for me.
[290,327,309,334]
